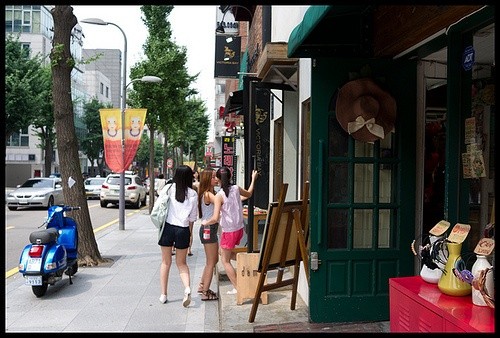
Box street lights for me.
[79,17,163,230]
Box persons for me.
[201,167,258,294]
[198,168,218,301]
[157,165,200,307]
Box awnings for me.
[286,5,348,58]
[222,90,243,120]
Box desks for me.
[232,208,267,253]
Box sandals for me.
[197,284,217,295]
[201,291,219,300]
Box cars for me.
[6,177,65,211]
[144,177,165,195]
[83,177,107,199]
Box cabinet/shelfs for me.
[389,276,495,333]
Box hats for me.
[336,79,397,142]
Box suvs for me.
[100,170,148,209]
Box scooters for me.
[19,202,82,298]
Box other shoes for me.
[183,288,191,307]
[188,253,193,255]
[226,288,238,295]
[172,252,177,255]
[160,297,168,304]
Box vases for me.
[420,236,448,283]
[437,243,472,296]
[471,254,494,306]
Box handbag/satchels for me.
[150,182,173,228]
[55,226,77,249]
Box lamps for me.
[216,5,252,33]
[226,123,234,132]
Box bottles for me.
[420,236,448,283]
[438,243,472,297]
[203,225,211,240]
[471,255,494,307]
